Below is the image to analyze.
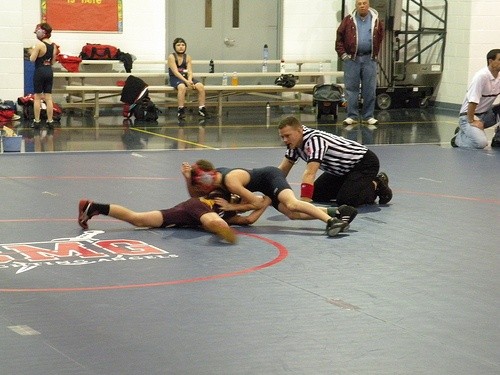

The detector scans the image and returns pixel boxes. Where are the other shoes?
[31,119,43,129]
[491,139,500,147]
[198,119,207,125]
[198,107,211,118]
[343,125,357,131]
[343,117,358,125]
[450,127,461,148]
[365,125,379,131]
[362,117,379,124]
[177,106,185,118]
[177,118,185,126]
[46,120,55,128]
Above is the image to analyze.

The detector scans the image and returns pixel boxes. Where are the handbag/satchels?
[274,73,296,88]
[80,42,119,61]
[135,98,159,121]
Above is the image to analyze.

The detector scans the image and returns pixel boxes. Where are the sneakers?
[338,204,358,224]
[326,218,350,237]
[78,200,93,230]
[375,172,393,204]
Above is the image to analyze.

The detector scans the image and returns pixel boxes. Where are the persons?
[30,23,57,130]
[335,0,384,124]
[276,116,393,206]
[167,37,209,120]
[181,160,357,236]
[77,190,272,243]
[451,50,500,149]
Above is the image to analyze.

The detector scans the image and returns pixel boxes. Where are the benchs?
[52,58,363,119]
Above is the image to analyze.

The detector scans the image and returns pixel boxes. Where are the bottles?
[231,71,239,86]
[280,60,285,73]
[265,102,271,116]
[208,59,214,73]
[263,45,269,60]
[262,61,267,72]
[318,62,323,72]
[222,72,227,85]
